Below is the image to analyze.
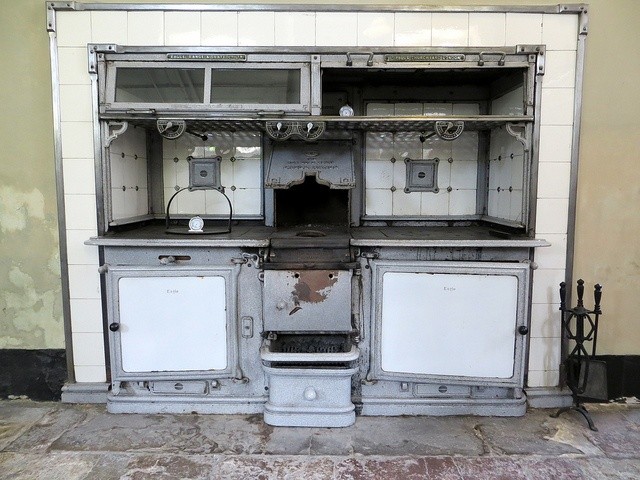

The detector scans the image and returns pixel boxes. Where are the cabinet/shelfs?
[371,255,526,396]
[106,262,246,388]
[316,58,539,125]
[96,53,313,124]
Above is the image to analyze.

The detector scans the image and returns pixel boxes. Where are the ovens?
[365,260,537,390]
[98,246,240,383]
